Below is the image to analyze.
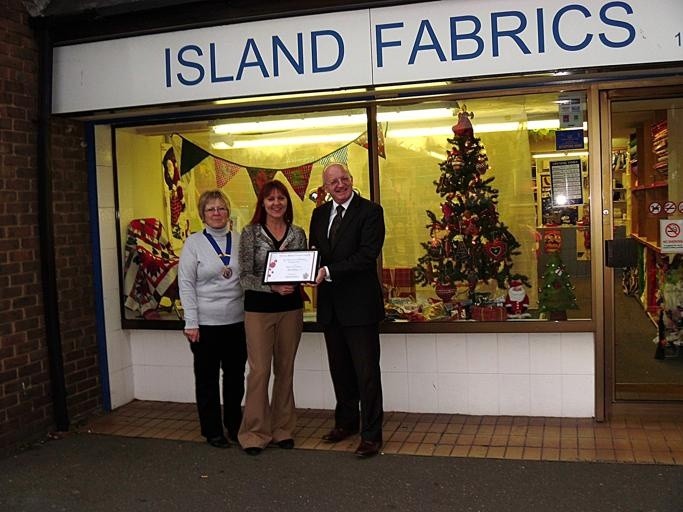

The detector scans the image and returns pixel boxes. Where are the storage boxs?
[382,268,416,302]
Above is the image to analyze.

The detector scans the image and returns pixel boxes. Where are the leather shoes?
[208,433,294,455]
[352,441,382,458]
[322,428,358,441]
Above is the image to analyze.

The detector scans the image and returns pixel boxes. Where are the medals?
[223,268,232,279]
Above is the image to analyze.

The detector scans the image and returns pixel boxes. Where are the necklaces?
[266,224,284,242]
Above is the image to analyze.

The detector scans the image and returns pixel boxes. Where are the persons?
[237,179,307,456]
[300,162,386,458]
[178,188,248,449]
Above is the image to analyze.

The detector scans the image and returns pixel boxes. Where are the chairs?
[121,217,185,318]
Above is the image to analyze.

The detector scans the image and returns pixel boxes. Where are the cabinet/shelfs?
[612,108,682,330]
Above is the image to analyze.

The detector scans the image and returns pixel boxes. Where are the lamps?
[207,100,461,136]
[532,150,589,159]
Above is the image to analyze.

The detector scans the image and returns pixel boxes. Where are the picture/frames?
[541,174,551,188]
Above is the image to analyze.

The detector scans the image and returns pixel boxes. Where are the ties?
[329,206,345,252]
